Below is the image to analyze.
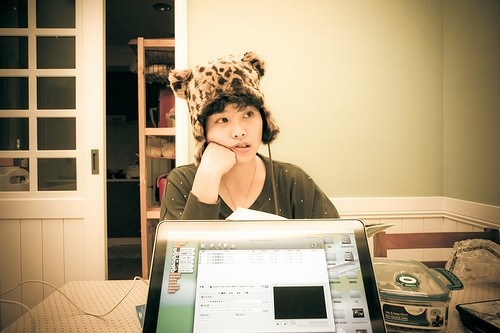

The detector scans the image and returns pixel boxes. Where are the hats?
[168,52,280,167]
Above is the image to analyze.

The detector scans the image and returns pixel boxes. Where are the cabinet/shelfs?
[106,65,161,122]
[128,36,176,278]
[107,182,141,238]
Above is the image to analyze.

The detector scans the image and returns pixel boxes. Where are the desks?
[0,281,500,333]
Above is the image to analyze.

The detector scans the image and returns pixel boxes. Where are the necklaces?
[222,159,257,210]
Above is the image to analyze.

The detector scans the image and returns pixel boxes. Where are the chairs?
[372,227,500,270]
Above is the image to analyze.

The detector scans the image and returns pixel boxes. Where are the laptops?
[140,217,388,333]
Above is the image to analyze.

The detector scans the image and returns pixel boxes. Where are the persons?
[158,50,340,220]
